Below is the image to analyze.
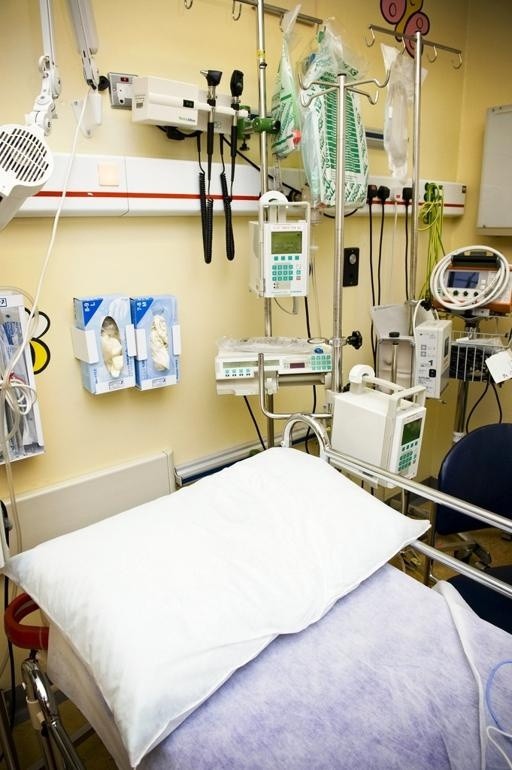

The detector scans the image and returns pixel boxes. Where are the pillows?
[0,445,433,770]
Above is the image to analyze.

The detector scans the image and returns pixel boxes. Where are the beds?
[0,408,512,770]
